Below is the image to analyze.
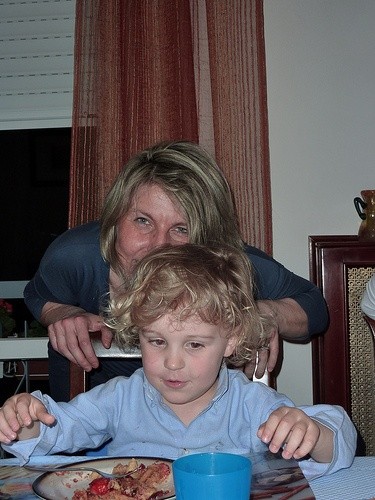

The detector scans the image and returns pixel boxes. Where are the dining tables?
[0,456,375,500]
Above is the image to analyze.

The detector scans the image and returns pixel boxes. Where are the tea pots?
[352,188,375,235]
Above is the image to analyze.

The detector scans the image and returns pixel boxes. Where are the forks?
[22,461,145,479]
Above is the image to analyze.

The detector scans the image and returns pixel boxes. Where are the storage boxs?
[69,338,270,399]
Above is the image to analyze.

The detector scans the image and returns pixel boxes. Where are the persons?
[0,243,357,480]
[23,141,329,402]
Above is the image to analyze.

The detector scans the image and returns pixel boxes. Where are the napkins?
[297,457,375,500]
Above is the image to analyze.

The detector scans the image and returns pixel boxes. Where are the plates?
[33,456,173,500]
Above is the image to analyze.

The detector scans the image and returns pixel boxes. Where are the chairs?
[0,281,49,394]
[308,234,375,457]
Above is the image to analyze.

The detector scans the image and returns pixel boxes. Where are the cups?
[170,451,255,498]
